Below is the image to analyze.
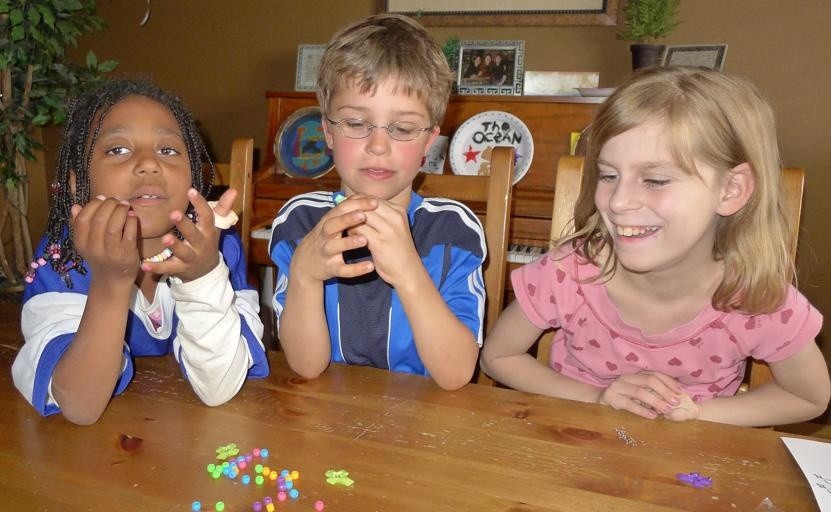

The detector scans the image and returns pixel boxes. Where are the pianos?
[251,90,610,346]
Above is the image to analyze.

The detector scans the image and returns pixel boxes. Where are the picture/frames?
[376,0,620,29]
[457,38,527,97]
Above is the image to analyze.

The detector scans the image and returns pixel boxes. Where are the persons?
[269,14,490,391]
[477,53,497,80]
[9,79,270,426]
[478,64,831,428]
[492,53,508,86]
[464,56,483,79]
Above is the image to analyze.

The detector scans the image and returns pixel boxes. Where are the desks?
[1,289,831,512]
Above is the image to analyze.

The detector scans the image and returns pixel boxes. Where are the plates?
[448,110,536,188]
[572,87,618,98]
[272,105,336,180]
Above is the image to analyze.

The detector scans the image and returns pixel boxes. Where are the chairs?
[340,146,516,386]
[537,155,807,431]
[197,137,255,282]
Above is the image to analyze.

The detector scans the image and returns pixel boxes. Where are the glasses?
[325,112,433,142]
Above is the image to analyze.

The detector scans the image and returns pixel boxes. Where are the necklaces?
[137,248,172,265]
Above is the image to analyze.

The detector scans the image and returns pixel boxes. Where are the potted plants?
[614,0,683,72]
[0,2,118,303]
